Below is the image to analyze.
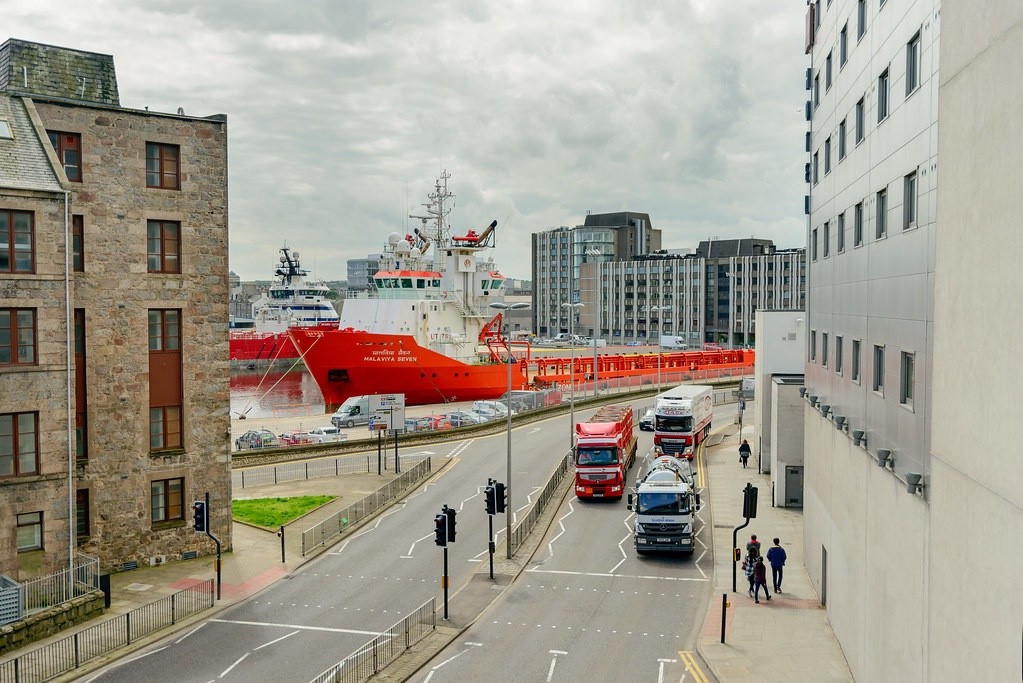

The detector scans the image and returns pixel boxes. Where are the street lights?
[651,306,667,393]
[490,303,530,559]
[561,302,585,448]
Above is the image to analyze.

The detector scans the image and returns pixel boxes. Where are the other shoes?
[778,588,782,593]
[748,590,752,598]
[767,595,771,600]
[755,601,759,603]
[774,587,777,593]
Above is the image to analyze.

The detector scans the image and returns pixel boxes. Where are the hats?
[744,439,747,442]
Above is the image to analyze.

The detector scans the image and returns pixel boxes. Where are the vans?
[498,390,545,410]
[331,396,368,428]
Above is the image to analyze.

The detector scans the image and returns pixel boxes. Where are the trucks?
[661,336,689,350]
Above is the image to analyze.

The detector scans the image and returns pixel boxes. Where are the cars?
[423,416,451,430]
[472,401,516,417]
[308,427,341,444]
[516,333,589,346]
[704,342,724,351]
[441,414,477,426]
[405,418,430,432]
[626,342,639,346]
[472,410,495,420]
[449,411,489,424]
[639,409,655,431]
[276,431,312,445]
[235,429,279,450]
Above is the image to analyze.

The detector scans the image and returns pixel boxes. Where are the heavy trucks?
[571,406,637,502]
[653,385,714,462]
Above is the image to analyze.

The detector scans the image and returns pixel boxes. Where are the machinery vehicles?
[627,455,700,558]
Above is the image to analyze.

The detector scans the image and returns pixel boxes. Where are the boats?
[285,169,755,405]
[229,239,339,368]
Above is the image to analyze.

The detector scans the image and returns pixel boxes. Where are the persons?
[744,549,757,597]
[754,556,771,603]
[579,451,590,459]
[766,538,787,593]
[746,535,761,557]
[738,439,751,468]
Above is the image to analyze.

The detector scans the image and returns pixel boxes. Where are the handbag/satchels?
[739,457,742,462]
[741,560,746,570]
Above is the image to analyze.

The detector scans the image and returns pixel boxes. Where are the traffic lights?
[191,501,206,533]
[484,483,508,515]
[434,514,448,548]
[443,508,457,543]
